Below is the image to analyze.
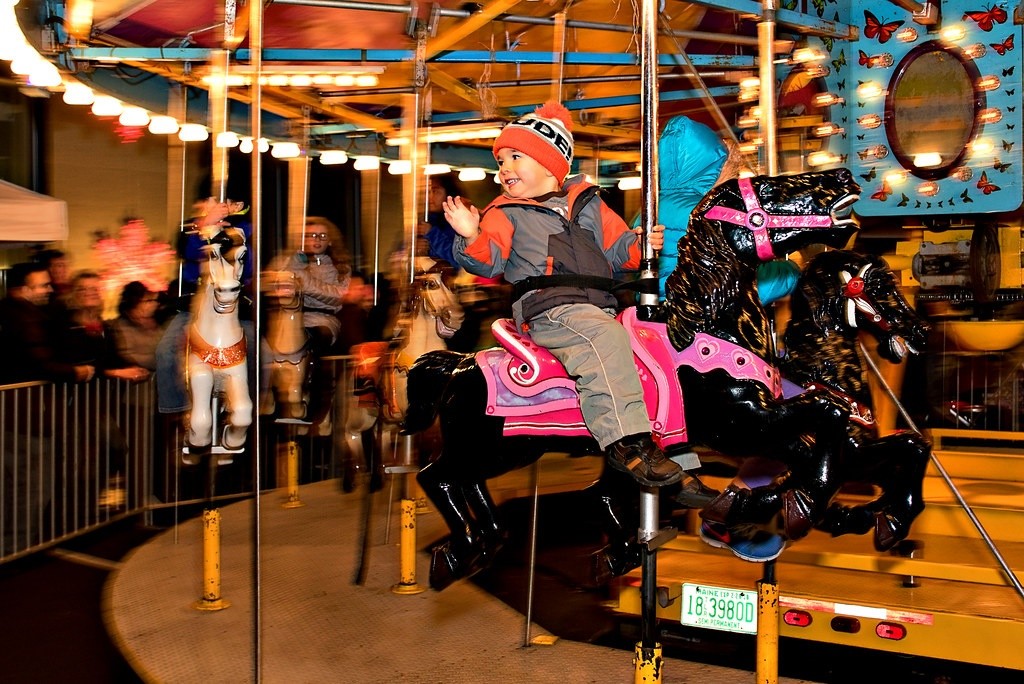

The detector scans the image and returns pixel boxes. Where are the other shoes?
[181,410,194,446]
[99,476,128,508]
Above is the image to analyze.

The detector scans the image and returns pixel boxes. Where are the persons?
[153,170,271,426]
[630,113,803,564]
[0,248,270,551]
[442,98,688,488]
[335,266,400,355]
[262,212,355,430]
[416,168,480,417]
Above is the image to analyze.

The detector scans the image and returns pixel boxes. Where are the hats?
[492,100,576,189]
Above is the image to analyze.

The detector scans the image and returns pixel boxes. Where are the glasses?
[304,233,329,241]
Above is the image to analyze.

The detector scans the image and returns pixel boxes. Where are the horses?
[396,168,863,594]
[661,248,935,554]
[257,249,468,495]
[155,228,254,466]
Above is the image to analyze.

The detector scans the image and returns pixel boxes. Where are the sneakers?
[659,467,717,507]
[408,496,430,515]
[602,431,682,487]
[699,515,787,562]
